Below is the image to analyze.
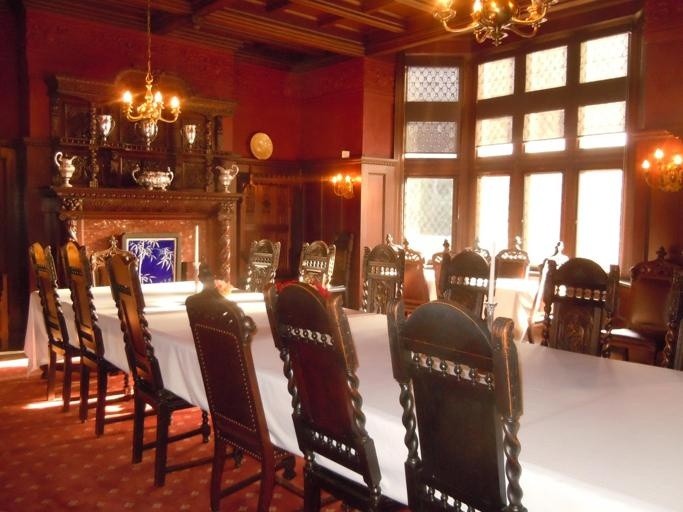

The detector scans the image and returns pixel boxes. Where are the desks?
[378,268,566,342]
[23,274,682,512]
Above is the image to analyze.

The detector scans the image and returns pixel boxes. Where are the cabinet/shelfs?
[46,71,239,188]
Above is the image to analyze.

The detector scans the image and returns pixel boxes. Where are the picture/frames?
[121,232,182,285]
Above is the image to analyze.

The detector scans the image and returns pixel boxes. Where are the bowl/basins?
[214,283,236,297]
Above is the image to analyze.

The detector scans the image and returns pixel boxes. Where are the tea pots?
[53,150,80,188]
[214,163,239,193]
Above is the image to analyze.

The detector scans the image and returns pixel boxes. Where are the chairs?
[358,242,407,320]
[329,237,353,311]
[519,242,570,344]
[245,237,282,294]
[104,248,242,487]
[600,245,682,368]
[184,288,297,512]
[665,308,665,311]
[539,257,619,360]
[439,250,490,326]
[388,237,531,278]
[61,237,156,438]
[386,299,526,512]
[28,241,131,413]
[262,282,382,512]
[296,240,336,293]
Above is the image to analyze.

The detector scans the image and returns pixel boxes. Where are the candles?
[194,223,199,263]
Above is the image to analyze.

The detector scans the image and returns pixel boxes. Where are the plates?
[249,132,274,160]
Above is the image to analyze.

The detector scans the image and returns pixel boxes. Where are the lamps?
[122,0,182,150]
[331,173,355,200]
[640,146,683,194]
[432,0,558,45]
